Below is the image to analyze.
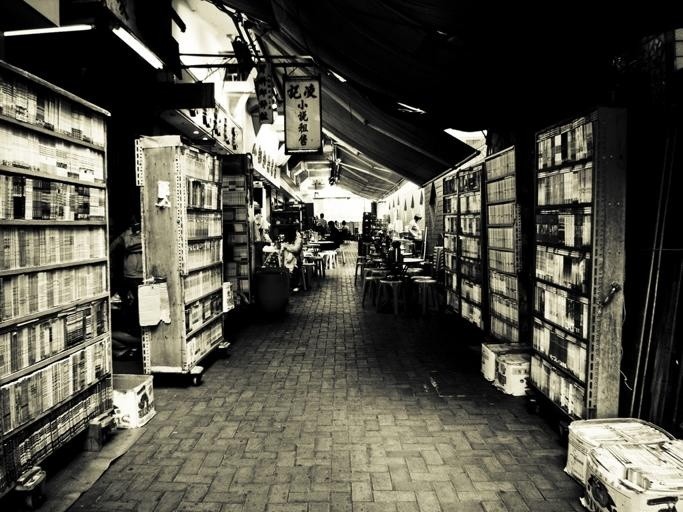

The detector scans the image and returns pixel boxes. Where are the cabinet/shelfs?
[215,150,255,318]
[444,105,629,421]
[3,58,116,505]
[133,133,229,382]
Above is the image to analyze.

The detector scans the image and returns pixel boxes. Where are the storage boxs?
[562,415,683,512]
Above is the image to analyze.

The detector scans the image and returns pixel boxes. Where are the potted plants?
[253,264,292,311]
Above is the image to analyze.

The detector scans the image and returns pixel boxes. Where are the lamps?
[111,23,167,71]
[3,24,96,37]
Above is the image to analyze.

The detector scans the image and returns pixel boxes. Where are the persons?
[255,211,351,295]
[112,211,143,351]
[367,212,425,271]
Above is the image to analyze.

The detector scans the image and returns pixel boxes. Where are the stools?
[293,231,440,321]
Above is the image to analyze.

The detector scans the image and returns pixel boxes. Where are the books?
[443,165,482,327]
[481,149,528,395]
[568,417,683,496]
[219,175,249,302]
[177,147,222,365]
[0,81,114,489]
[530,117,593,419]
